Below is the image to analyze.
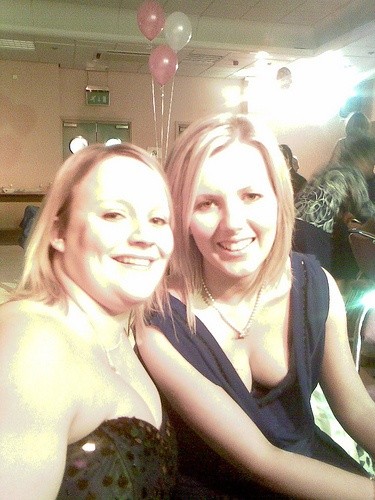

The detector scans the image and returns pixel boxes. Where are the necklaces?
[192,257,263,339]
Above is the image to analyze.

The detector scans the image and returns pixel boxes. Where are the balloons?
[149,44,177,86]
[137,1,164,44]
[163,11,194,54]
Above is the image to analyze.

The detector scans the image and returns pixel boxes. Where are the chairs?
[348,226,375,375]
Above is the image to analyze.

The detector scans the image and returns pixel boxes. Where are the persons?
[322,113,372,271]
[134,113,375,500]
[0,142,184,500]
[290,135,375,272]
[288,154,300,176]
[276,142,311,202]
